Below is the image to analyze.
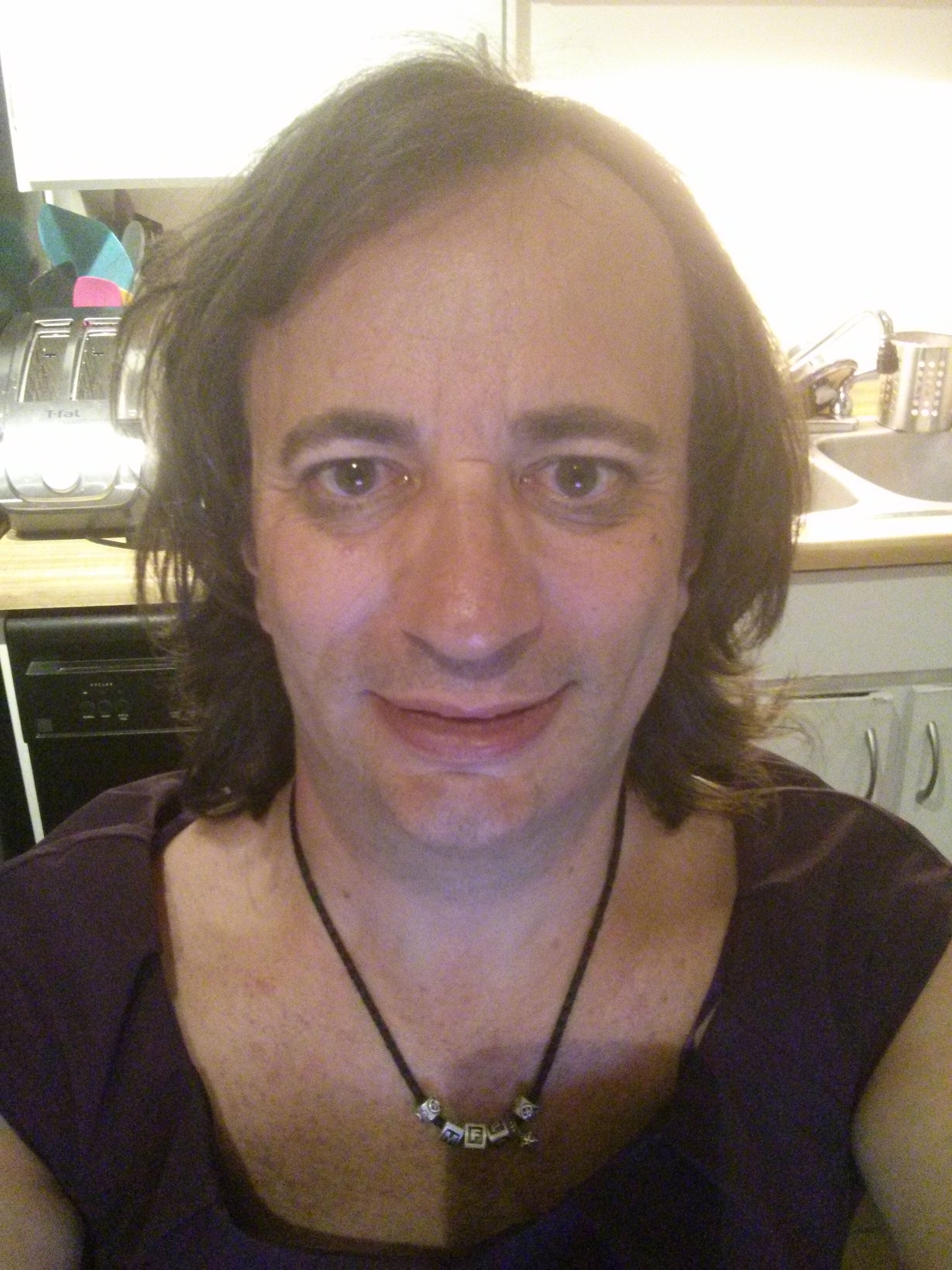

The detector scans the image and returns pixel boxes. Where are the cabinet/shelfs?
[2,1,520,193]
[727,684,952,864]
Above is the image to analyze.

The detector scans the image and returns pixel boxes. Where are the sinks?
[801,461,859,513]
[817,418,952,502]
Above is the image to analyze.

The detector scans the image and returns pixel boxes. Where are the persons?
[0,45,952,1270]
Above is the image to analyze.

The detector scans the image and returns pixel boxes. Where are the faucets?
[771,305,899,434]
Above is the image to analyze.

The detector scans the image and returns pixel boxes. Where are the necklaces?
[286,769,626,1147]
